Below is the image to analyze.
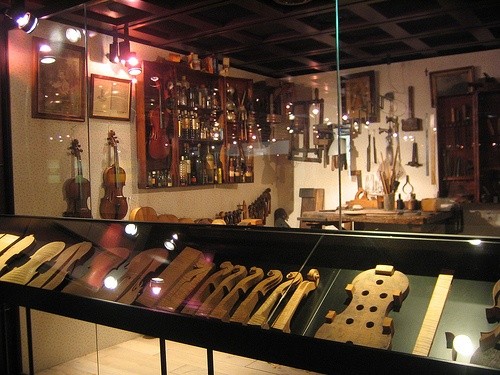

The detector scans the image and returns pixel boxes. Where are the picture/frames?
[340,70,379,123]
[91,74,132,121]
[31,36,85,122]
[429,66,474,107]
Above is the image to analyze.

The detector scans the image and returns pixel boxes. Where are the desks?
[302,200,457,234]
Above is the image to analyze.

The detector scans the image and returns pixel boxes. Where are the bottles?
[146,169,172,188]
[397,194,403,209]
[178,75,253,185]
[408,194,417,211]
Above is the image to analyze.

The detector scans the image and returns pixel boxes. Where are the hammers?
[401,86,419,132]
[408,143,421,167]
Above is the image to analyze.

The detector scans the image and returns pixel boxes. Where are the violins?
[99,129,129,219]
[146,77,170,159]
[64,139,94,219]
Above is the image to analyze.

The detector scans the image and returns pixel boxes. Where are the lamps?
[110,22,142,75]
[0,0,39,34]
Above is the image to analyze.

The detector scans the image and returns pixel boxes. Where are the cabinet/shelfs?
[435,92,500,204]
[136,59,254,189]
